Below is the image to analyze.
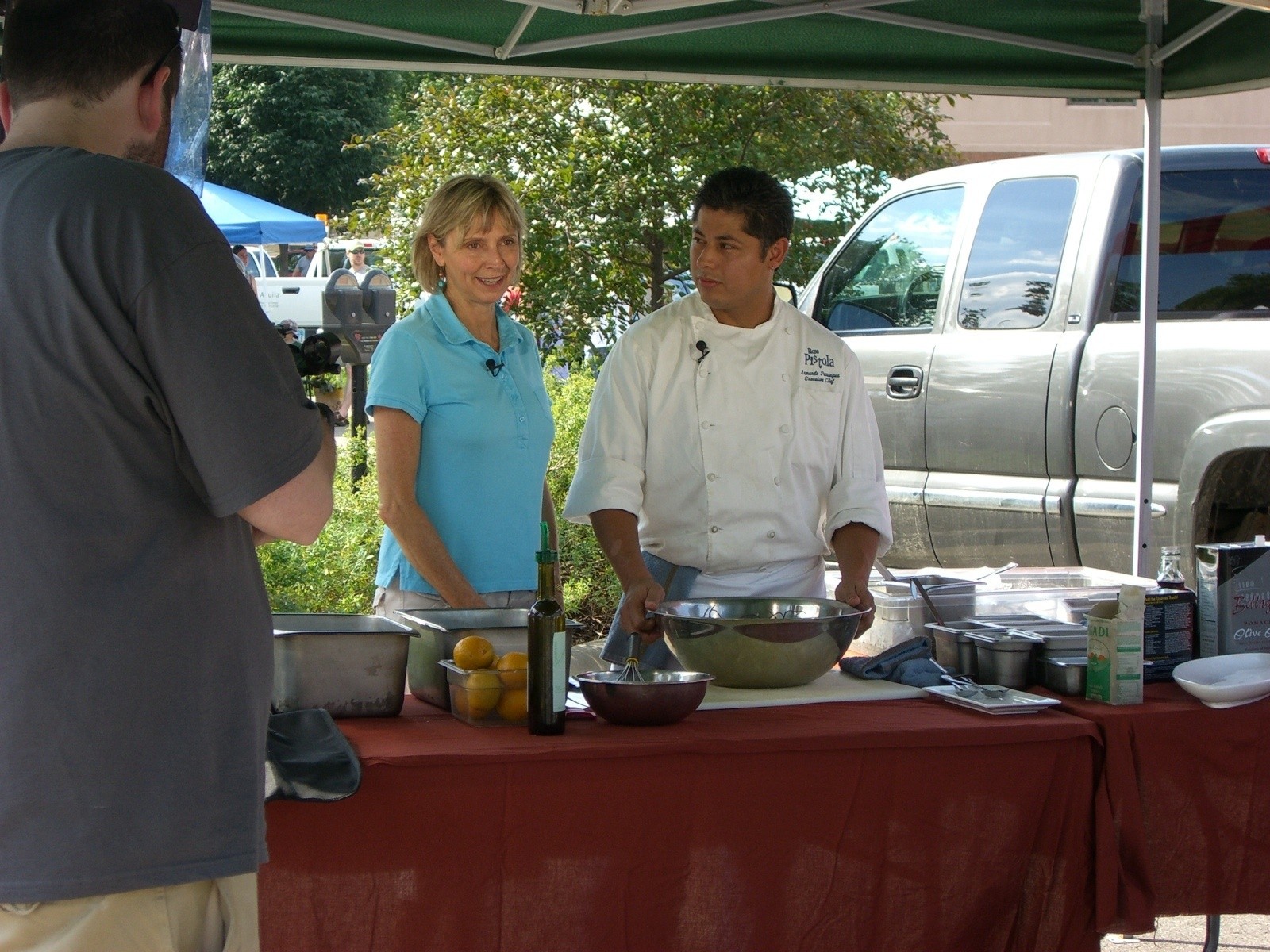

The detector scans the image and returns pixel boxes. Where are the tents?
[170,174,327,277]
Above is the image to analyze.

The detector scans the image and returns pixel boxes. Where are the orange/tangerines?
[453,636,529,720]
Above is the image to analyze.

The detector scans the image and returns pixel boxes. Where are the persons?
[364,173,555,626]
[232,244,258,299]
[562,165,893,671]
[0,0,337,952]
[275,319,308,377]
[292,244,317,277]
[342,240,375,289]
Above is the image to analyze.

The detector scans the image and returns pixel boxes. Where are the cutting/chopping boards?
[696,669,928,709]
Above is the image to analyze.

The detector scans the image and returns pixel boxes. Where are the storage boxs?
[1083,584,1148,703]
[254,277,337,325]
[269,612,421,716]
[395,605,586,710]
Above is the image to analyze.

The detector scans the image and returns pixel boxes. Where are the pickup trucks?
[770,141,1269,601]
[228,235,398,375]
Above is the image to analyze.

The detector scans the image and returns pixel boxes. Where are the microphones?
[486,359,496,376]
[696,340,706,357]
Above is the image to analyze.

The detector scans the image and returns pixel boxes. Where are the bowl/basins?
[574,671,717,725]
[646,597,872,689]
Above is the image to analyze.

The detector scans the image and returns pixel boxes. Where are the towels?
[263,708,361,803]
[839,634,975,690]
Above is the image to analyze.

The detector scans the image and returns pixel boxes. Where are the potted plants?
[304,373,344,412]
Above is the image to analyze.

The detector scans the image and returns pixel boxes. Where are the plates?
[1173,652,1270,710]
[922,683,1062,715]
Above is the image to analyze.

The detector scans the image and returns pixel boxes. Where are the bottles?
[528,520,568,736]
[1157,546,1185,590]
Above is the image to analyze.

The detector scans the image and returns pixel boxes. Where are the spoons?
[930,658,1010,698]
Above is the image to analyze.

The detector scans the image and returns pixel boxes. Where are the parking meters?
[320,264,401,491]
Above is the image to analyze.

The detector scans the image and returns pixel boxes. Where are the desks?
[259,661,1097,952]
[1025,683,1270,952]
